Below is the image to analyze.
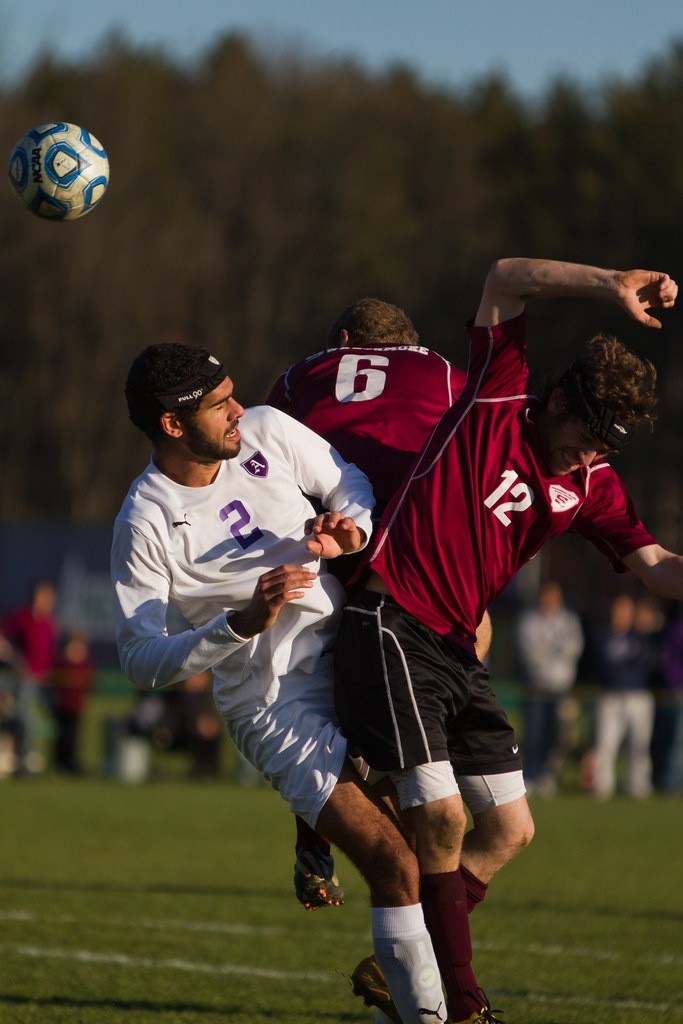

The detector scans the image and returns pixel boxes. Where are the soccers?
[7,121,110,221]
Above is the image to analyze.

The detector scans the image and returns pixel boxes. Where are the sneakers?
[293,852,344,910]
[448,987,503,1024]
[351,956,399,1024]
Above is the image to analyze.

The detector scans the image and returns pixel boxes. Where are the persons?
[1,583,92,779]
[108,259,683,1024]
